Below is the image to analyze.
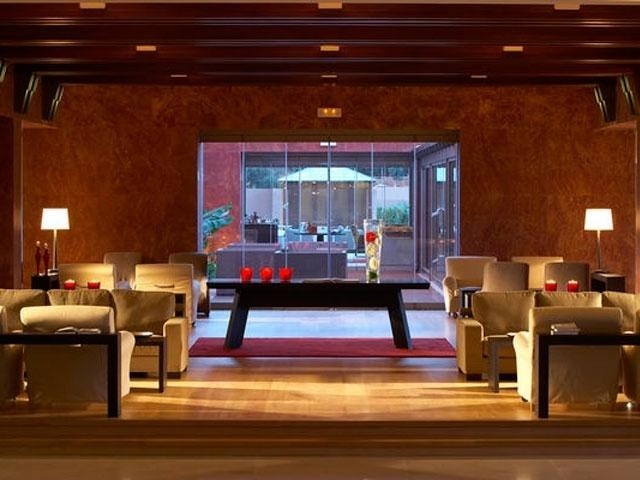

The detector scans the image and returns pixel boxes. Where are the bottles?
[34,241,42,276]
[42,242,50,276]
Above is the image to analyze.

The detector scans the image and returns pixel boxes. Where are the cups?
[544,282,557,291]
[299,221,318,234]
[568,281,579,292]
[64,282,76,290]
[362,219,383,279]
[240,268,252,280]
[87,281,100,289]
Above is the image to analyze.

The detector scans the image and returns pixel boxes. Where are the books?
[551,322,579,335]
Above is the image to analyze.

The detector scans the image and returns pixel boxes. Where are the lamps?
[40,207,71,272]
[585,207,613,272]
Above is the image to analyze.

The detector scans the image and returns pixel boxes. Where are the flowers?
[364,231,381,282]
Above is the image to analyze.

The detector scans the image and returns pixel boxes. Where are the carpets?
[189,336,456,358]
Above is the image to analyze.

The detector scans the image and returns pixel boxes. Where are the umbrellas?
[276,167,377,183]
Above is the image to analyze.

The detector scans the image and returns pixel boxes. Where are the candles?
[240,266,252,281]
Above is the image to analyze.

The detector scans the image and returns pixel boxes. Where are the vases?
[363,219,382,282]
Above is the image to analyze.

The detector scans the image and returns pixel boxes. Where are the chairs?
[351,226,366,256]
[442,256,639,420]
[0,249,208,418]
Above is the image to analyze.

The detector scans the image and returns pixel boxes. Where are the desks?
[207,278,429,348]
[293,227,346,242]
[245,223,278,245]
